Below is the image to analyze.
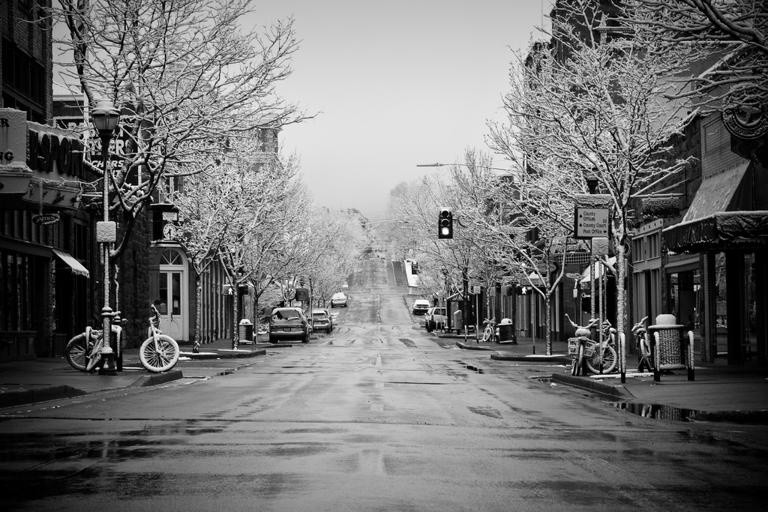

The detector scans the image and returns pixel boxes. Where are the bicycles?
[66,305,130,372]
[560,311,653,377]
[139,302,181,372]
[479,315,496,342]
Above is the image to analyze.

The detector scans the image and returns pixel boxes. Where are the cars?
[340,281,350,289]
[413,298,430,315]
[307,319,313,335]
[330,291,349,308]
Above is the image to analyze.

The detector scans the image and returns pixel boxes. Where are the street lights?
[85,94,126,372]
[416,163,537,356]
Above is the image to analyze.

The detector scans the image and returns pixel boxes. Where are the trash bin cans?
[496,318,514,344]
[239,319,254,344]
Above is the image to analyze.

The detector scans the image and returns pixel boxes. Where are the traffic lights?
[223,284,235,296]
[438,208,454,239]
[516,284,532,295]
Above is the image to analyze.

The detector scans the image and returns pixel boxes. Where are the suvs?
[422,306,448,331]
[309,308,334,335]
[268,307,310,344]
